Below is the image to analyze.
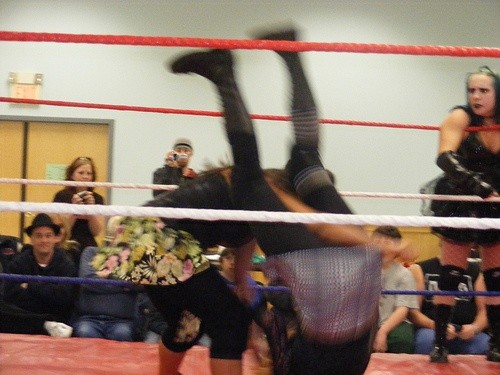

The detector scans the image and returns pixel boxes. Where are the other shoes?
[167,49,235,77]
[252,27,298,52]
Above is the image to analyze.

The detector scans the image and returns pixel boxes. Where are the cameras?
[174,154,188,163]
[77,192,87,202]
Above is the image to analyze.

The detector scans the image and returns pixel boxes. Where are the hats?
[172,136,194,154]
[25,212,61,237]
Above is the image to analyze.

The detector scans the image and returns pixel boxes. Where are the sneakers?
[50,322,73,339]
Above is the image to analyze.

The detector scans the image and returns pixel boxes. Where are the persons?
[167,26,381,375]
[429,66,500,361]
[0,144,490,375]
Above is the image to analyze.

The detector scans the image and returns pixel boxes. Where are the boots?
[428,303,453,364]
[482,302,500,362]
